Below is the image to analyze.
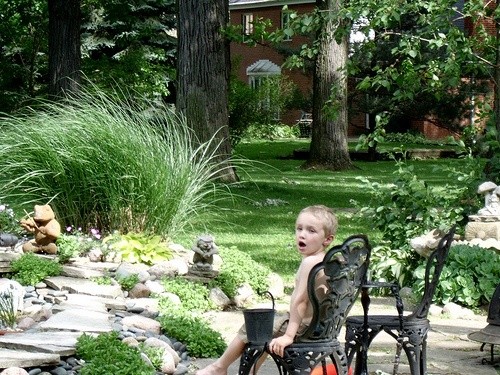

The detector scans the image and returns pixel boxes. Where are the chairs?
[344,224,456,375]
[239,235,372,375]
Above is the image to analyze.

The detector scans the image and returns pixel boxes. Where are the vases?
[241,308,276,346]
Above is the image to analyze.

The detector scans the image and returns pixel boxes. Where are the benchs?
[402,149,457,160]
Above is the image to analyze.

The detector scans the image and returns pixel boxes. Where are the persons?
[195,204,345,375]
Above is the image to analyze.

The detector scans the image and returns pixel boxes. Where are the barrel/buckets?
[242,288,276,346]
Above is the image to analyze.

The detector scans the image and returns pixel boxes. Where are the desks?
[321,282,404,375]
